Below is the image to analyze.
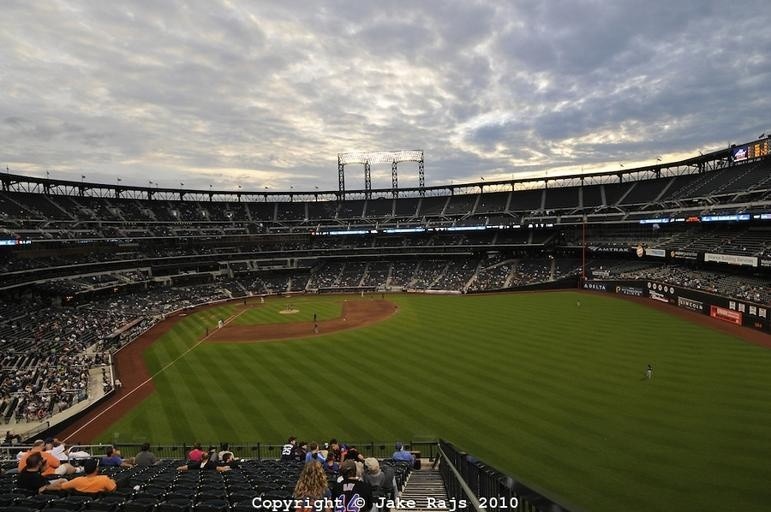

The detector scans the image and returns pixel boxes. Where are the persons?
[0,193,771,512]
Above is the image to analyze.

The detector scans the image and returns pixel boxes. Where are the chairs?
[0,457,410,512]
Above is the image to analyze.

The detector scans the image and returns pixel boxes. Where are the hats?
[364,457,379,471]
[341,459,357,477]
